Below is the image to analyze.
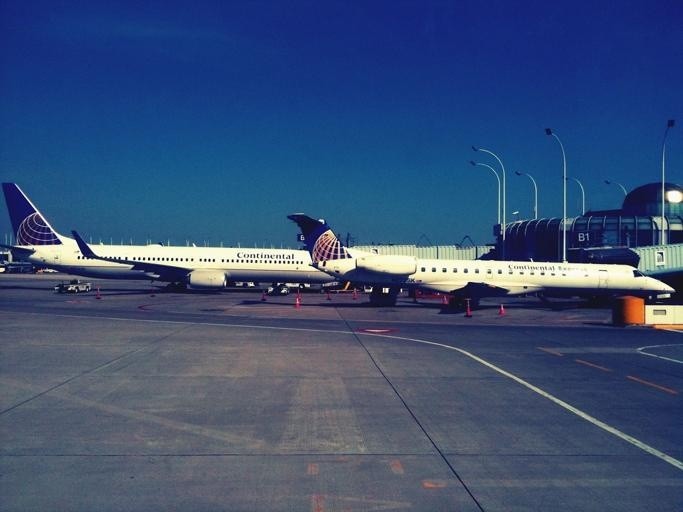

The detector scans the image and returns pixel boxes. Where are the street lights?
[470,145,506,241]
[562,176,585,216]
[661,119,674,247]
[604,180,627,197]
[544,127,567,261]
[514,170,537,220]
